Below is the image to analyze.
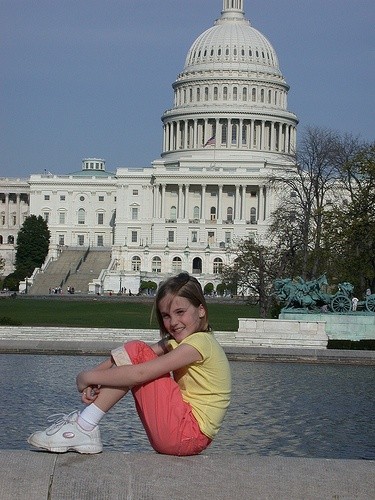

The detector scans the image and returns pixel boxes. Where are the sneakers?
[27,409,103,454]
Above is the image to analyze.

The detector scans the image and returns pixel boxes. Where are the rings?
[83,392,86,395]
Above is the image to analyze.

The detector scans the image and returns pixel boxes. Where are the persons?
[27,273,231,455]
[49,285,244,298]
